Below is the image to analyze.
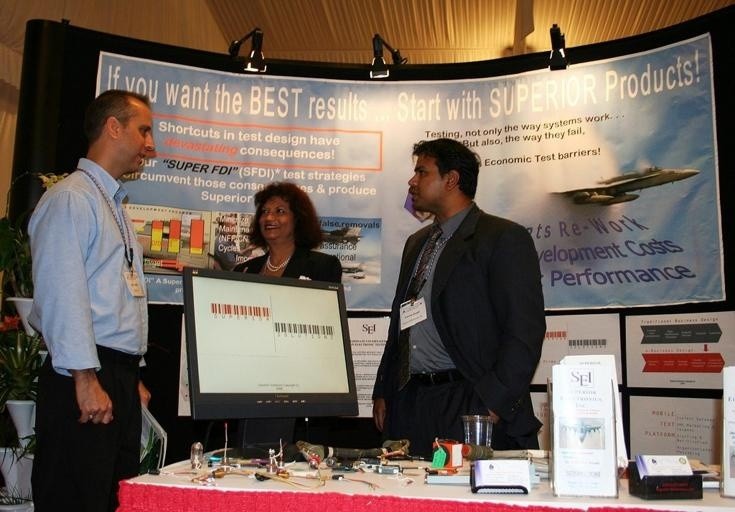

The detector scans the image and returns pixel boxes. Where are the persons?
[230,181,344,461]
[371,137,548,461]
[24,91,157,512]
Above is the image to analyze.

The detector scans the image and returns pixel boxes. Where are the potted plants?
[1,213,43,511]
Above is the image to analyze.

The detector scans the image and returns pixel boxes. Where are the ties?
[393,223,444,393]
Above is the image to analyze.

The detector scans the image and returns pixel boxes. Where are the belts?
[404,368,468,389]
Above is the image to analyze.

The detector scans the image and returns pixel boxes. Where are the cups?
[461,412,494,447]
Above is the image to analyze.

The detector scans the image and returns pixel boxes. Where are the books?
[139,402,167,475]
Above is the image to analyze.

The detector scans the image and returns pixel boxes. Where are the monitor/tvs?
[183,266,358,462]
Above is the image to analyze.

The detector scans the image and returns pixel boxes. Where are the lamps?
[543,21,572,75]
[365,31,407,78]
[228,23,271,76]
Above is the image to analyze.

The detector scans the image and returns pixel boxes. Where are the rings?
[88,414,94,419]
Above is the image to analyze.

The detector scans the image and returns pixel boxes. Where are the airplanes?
[323,225,362,244]
[550,166,700,206]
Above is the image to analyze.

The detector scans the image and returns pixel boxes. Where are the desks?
[117,444,735,512]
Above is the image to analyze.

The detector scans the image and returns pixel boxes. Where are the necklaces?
[266,254,291,272]
